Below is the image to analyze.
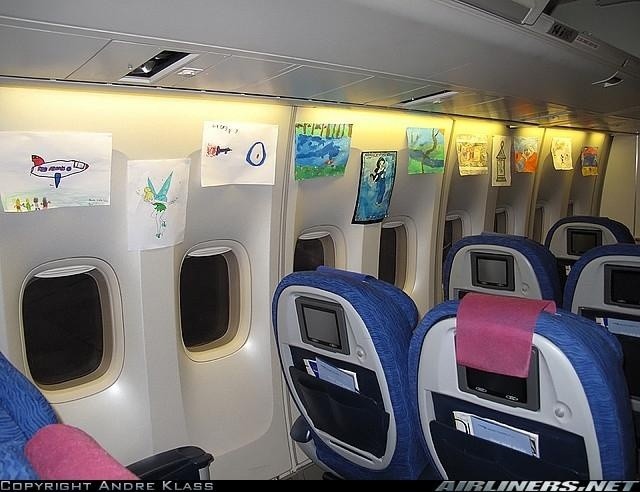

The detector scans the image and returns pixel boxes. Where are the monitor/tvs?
[295,298,348,355]
[456,335,542,411]
[604,263,640,309]
[567,228,601,255]
[470,252,515,293]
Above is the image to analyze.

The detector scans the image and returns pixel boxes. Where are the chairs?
[564,246,640,419]
[408,292,640,479]
[442,231,565,307]
[0,353,211,479]
[270,261,432,480]
[545,216,633,280]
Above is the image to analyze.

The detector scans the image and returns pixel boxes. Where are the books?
[303,358,360,393]
[452,410,541,459]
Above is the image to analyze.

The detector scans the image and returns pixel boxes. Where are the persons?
[144,187,168,238]
[369,157,388,208]
